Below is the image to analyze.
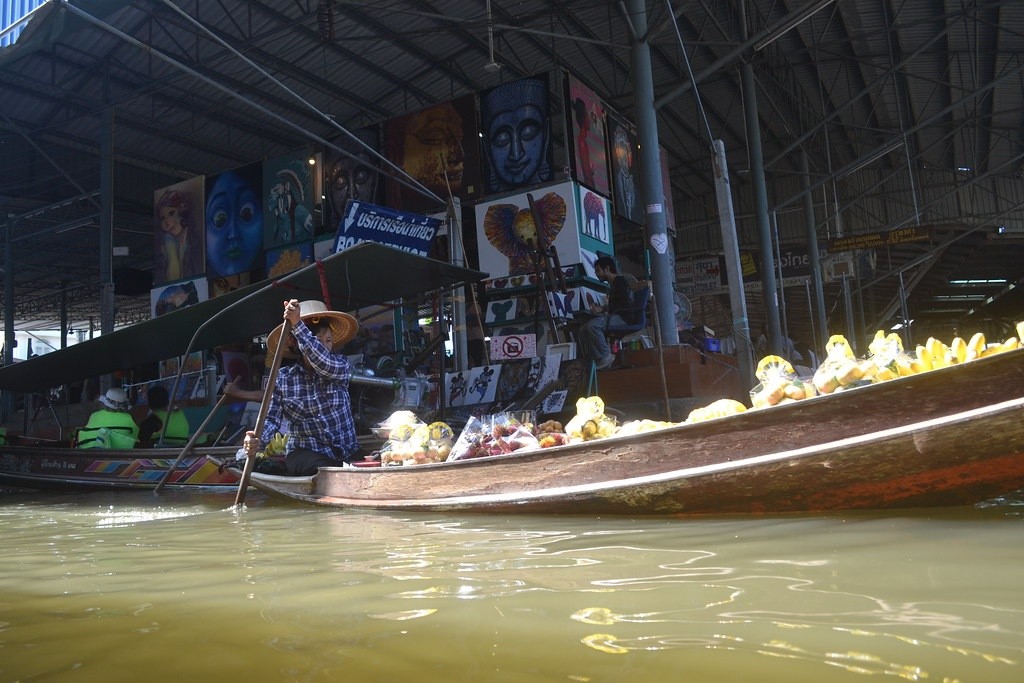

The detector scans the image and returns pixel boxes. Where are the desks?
[590,344,739,404]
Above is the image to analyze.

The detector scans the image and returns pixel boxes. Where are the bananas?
[881,333,1018,381]
[265,432,289,458]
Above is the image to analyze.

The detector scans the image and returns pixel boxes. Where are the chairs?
[605,287,651,353]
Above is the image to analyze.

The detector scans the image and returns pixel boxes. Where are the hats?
[99,388,132,409]
[266,300,359,359]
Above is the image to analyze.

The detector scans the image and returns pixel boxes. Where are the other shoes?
[596,353,615,370]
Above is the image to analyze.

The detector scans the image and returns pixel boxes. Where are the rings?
[290,305,296,311]
[247,442,253,449]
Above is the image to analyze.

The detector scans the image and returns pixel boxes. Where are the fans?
[673,291,692,327]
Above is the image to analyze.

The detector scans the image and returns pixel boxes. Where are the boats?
[206,342,1024,522]
[0,239,491,502]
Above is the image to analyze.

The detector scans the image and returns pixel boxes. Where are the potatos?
[539,420,563,433]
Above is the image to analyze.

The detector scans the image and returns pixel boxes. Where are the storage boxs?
[678,325,715,337]
[704,338,720,352]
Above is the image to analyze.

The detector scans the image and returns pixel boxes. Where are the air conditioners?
[829,259,856,281]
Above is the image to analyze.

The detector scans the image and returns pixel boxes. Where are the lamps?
[752,0,834,53]
[54,217,97,234]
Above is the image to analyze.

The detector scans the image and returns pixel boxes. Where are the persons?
[243,300,362,476]
[579,257,640,370]
[75,387,140,448]
[757,322,820,369]
[223,382,265,402]
[135,386,189,444]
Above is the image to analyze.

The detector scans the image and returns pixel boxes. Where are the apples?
[761,359,885,407]
[381,445,449,466]
